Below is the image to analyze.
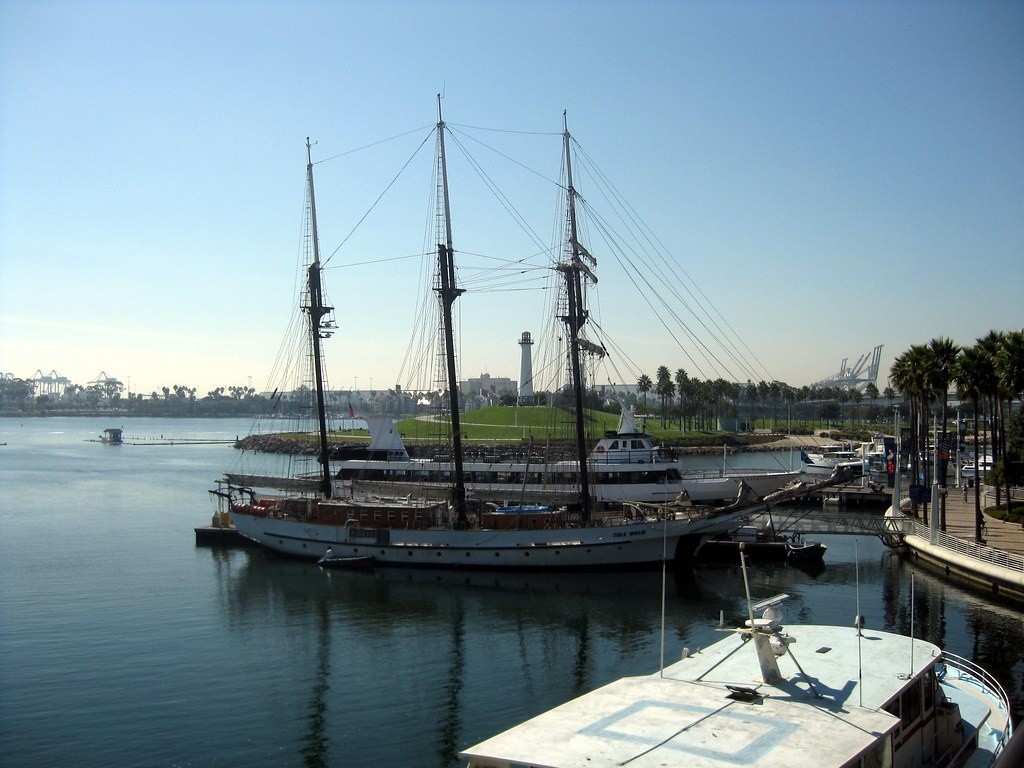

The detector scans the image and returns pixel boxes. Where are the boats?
[441,534,1020,766]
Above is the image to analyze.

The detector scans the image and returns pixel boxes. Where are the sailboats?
[208,93,996,583]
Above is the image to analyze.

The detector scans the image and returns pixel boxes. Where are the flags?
[882,437,895,489]
[900,427,911,456]
[959,421,968,435]
[801,449,815,464]
[937,431,952,487]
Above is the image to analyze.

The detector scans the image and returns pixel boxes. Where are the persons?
[980,509,985,529]
[961,482,968,499]
